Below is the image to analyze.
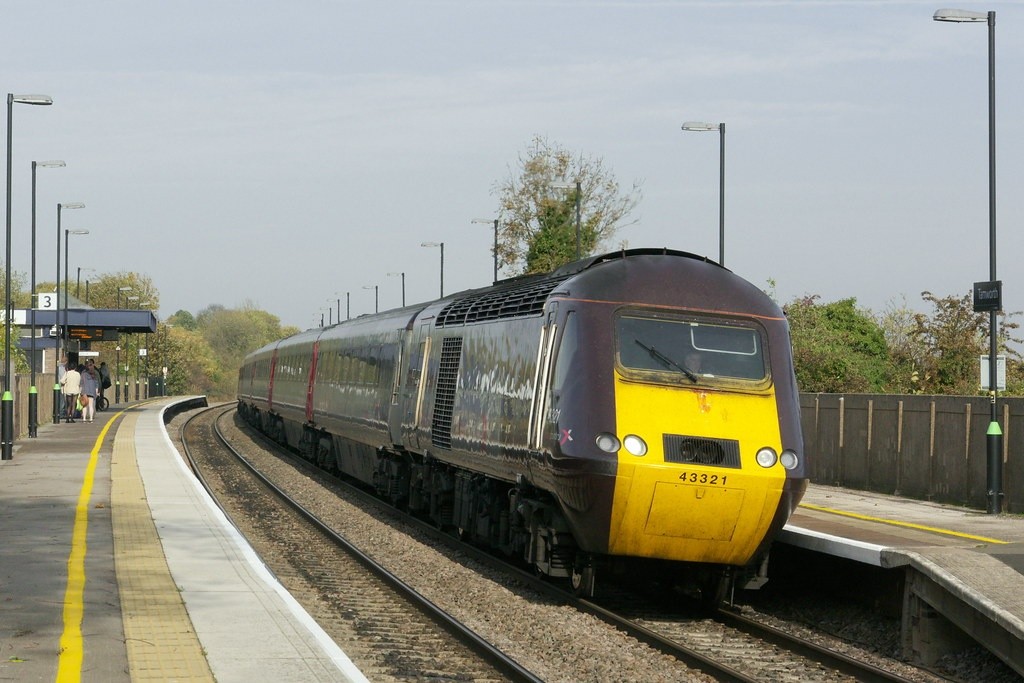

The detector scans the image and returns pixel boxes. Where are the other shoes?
[66,420,69,423]
[84,421,87,424]
[71,420,75,423]
[90,421,93,423]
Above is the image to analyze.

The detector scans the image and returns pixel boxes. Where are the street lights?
[548,180,580,260]
[387,273,405,308]
[683,120,725,267]
[2,94,54,459]
[63,229,89,357]
[156,320,174,397]
[29,160,66,438]
[135,303,150,400]
[932,7,1005,515]
[471,217,498,282]
[53,202,86,423]
[116,287,132,405]
[76,265,102,304]
[421,241,444,299]
[362,285,378,313]
[125,296,139,402]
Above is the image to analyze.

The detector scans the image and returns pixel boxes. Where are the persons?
[59,357,68,418]
[80,359,101,423]
[684,352,704,373]
[97,362,109,411]
[61,362,80,423]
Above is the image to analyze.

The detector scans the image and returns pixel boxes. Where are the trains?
[237,247,806,608]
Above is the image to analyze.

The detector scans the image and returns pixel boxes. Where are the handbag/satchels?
[76,394,83,411]
[80,393,89,407]
